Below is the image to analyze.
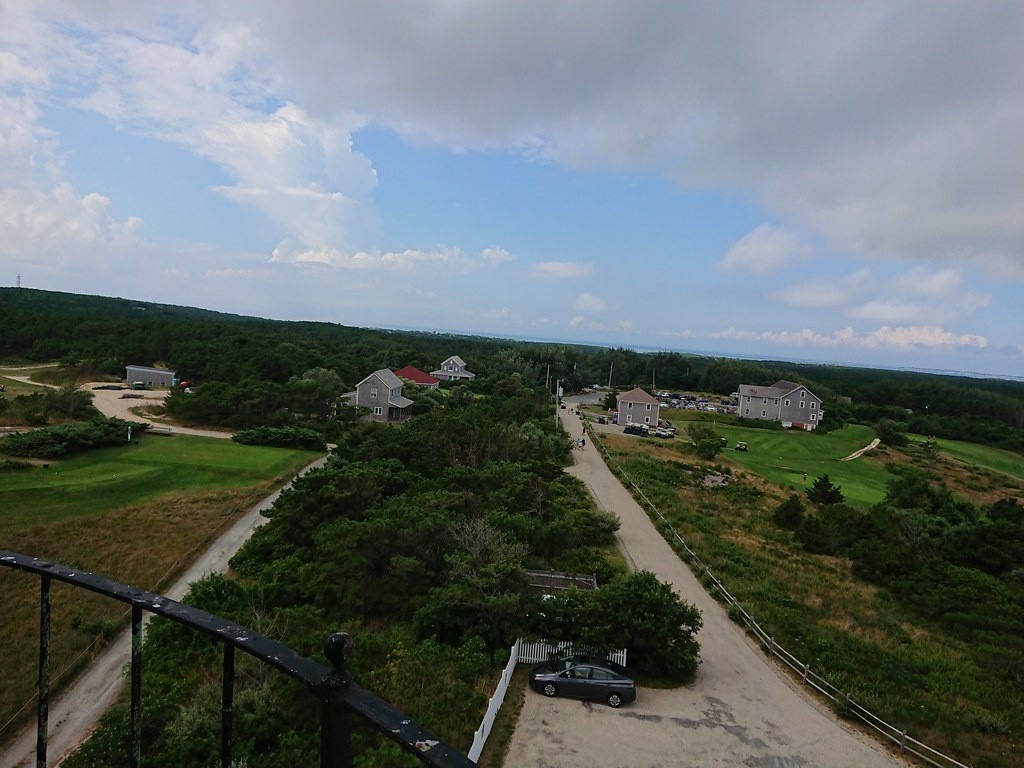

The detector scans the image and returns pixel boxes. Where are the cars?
[155,363,169,371]
[184,388,193,394]
[130,381,144,390]
[656,391,739,414]
[529,655,637,708]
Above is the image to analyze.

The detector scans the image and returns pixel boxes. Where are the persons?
[804,474,807,484]
[574,438,586,451]
[582,427,586,435]
